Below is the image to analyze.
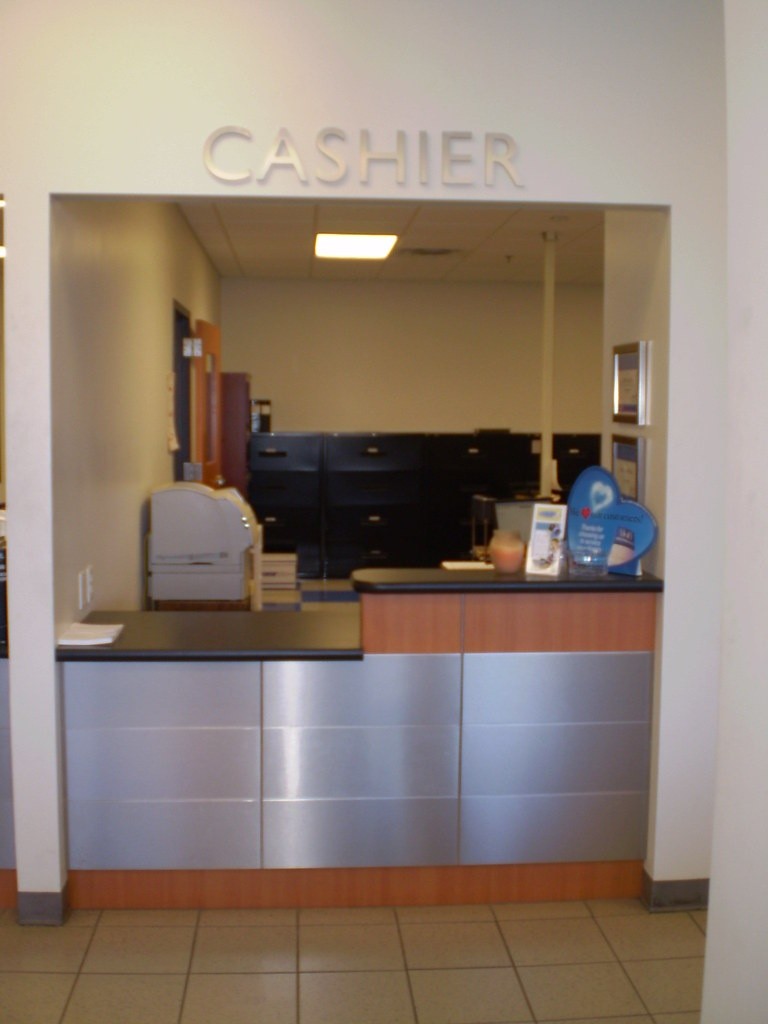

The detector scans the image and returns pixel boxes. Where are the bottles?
[490,529,525,574]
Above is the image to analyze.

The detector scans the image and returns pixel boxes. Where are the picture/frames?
[611,341,645,427]
[611,433,645,503]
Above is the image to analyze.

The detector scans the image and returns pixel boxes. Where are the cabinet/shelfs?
[248,432,600,580]
[220,369,253,497]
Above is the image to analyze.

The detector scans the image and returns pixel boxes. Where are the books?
[58,622,124,646]
